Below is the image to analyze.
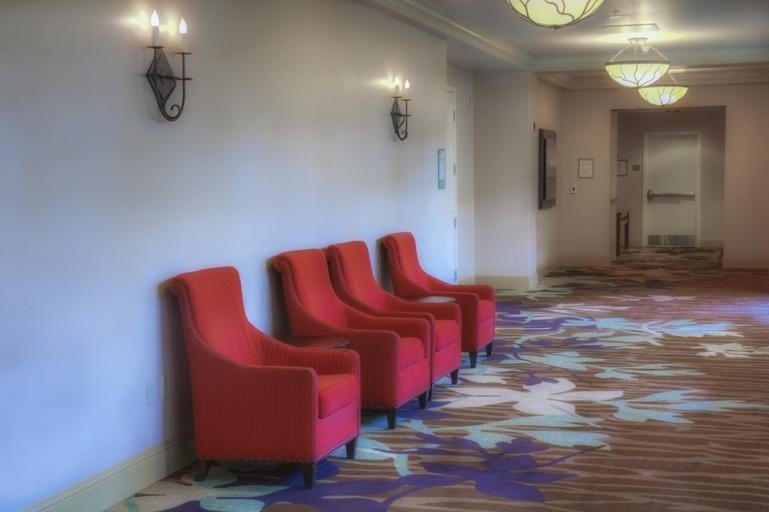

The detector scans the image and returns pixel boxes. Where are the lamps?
[147,9,189,123]
[390,78,410,141]
[505,0,604,30]
[605,37,689,107]
